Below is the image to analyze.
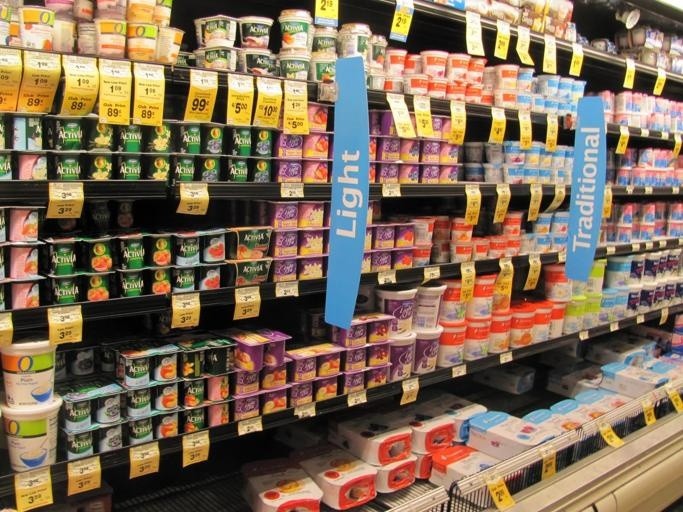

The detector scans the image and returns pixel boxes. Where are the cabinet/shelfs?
[0,1,682,510]
